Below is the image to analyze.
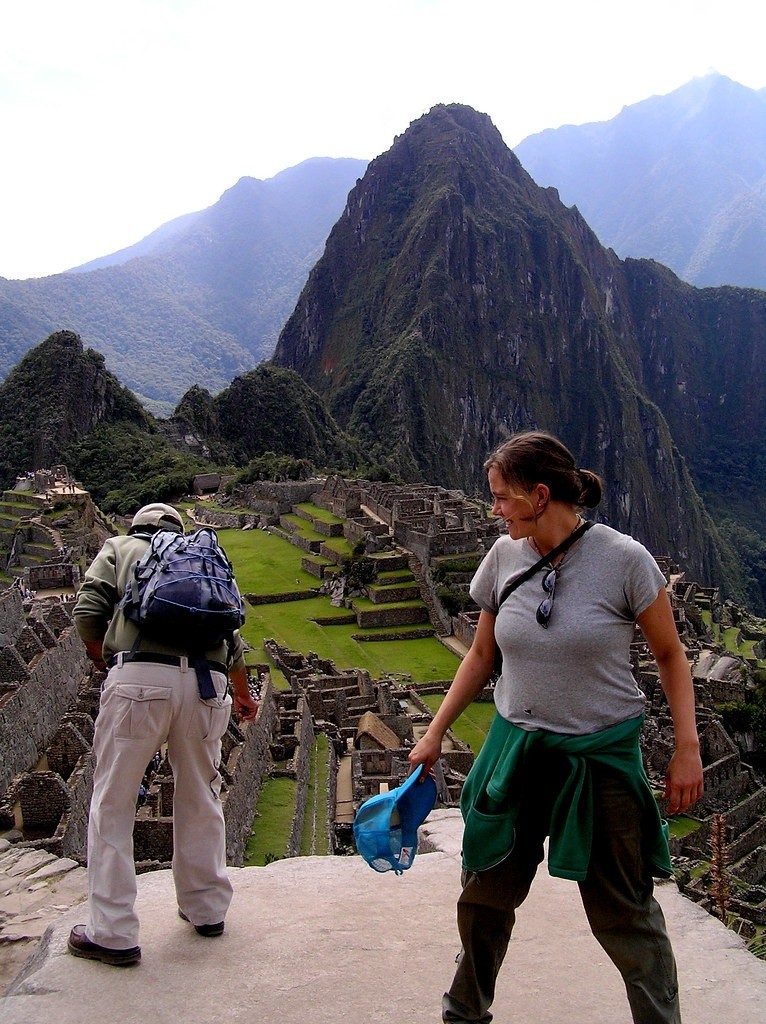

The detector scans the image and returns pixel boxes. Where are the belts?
[111,652,228,676]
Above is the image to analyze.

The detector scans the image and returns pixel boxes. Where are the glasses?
[536,569,557,629]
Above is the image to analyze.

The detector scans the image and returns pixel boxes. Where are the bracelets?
[86,651,103,663]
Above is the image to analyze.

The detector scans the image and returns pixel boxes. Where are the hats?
[353,764,437,876]
[127,503,185,534]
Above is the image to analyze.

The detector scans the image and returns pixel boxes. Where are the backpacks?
[114,526,246,650]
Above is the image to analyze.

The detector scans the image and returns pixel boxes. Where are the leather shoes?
[68,924,142,964]
[179,907,225,936]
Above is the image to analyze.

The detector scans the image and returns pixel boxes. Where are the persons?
[66,502,258,963]
[18,588,37,599]
[409,432,705,1024]
[137,751,161,805]
[60,592,73,601]
[246,673,262,701]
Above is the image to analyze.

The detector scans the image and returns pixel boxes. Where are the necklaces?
[533,514,581,569]
